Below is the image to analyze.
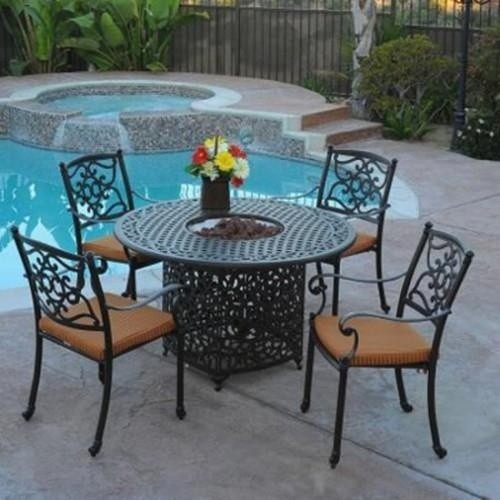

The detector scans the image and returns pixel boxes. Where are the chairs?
[300,221,474,468]
[11,226,185,457]
[59,149,162,304]
[271,145,397,315]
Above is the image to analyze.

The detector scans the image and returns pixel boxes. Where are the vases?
[201,174,229,210]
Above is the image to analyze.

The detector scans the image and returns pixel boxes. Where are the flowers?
[185,135,250,187]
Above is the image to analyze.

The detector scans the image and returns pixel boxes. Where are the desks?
[114,198,357,391]
